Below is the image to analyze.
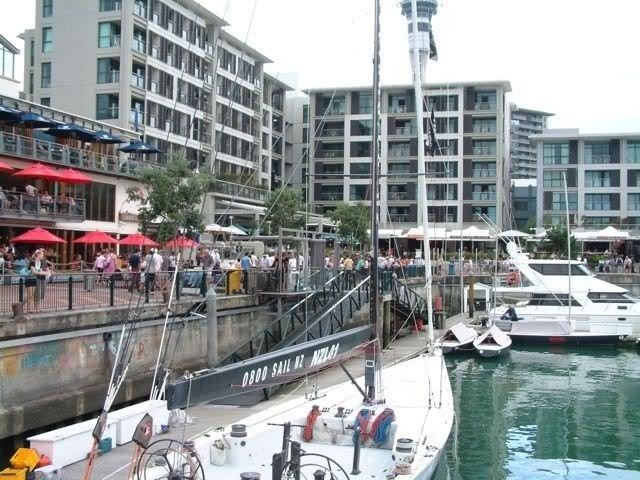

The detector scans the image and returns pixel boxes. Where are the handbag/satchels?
[19,260,35,276]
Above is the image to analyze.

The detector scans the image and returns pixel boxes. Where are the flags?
[426,119,438,158]
[430,106,437,133]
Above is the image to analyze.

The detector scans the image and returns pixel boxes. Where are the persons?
[73,247,183,303]
[0,182,81,217]
[325,255,334,267]
[448,254,513,272]
[236,242,304,292]
[597,249,636,273]
[377,254,426,278]
[1,242,54,311]
[339,253,370,284]
[196,247,221,297]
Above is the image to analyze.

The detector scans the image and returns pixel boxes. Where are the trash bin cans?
[434,312,447,329]
[82,269,98,292]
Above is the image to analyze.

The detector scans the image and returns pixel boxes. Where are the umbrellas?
[0,104,164,200]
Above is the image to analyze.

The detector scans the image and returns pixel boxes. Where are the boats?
[473,323,513,357]
[436,322,477,355]
[80,0,457,480]
[476,172,640,346]
